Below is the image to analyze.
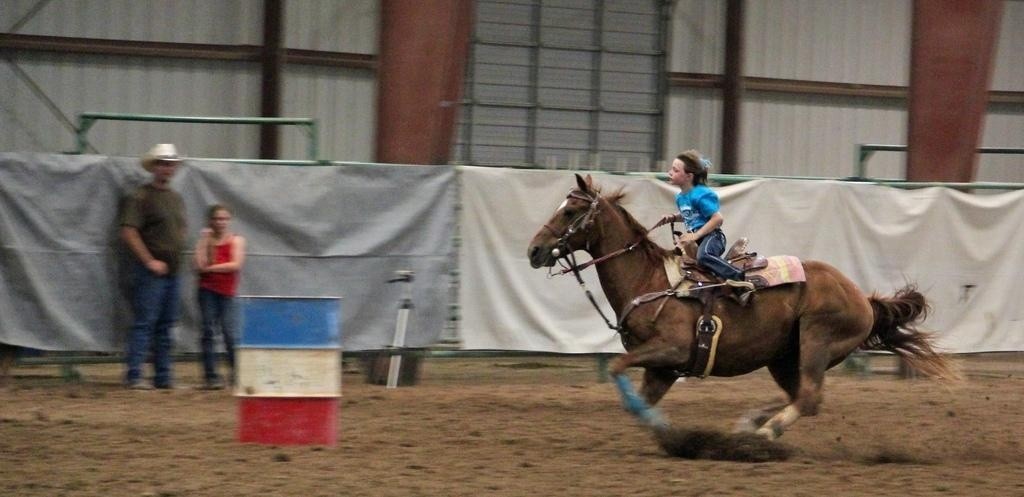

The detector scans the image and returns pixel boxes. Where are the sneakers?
[731,273,751,307]
[126,380,224,391]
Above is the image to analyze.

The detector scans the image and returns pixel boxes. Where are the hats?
[143,144,186,173]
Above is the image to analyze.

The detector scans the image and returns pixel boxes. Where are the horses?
[525,171,962,444]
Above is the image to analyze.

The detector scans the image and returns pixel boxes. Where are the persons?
[668,150,752,308]
[193,205,246,388]
[119,143,191,391]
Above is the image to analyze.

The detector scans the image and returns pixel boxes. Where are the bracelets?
[671,214,677,222]
